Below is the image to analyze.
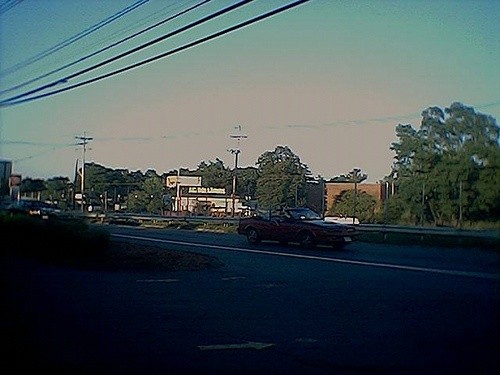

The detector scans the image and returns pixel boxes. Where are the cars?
[4,198,58,223]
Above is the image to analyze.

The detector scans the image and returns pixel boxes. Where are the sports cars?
[237,206,362,251]
